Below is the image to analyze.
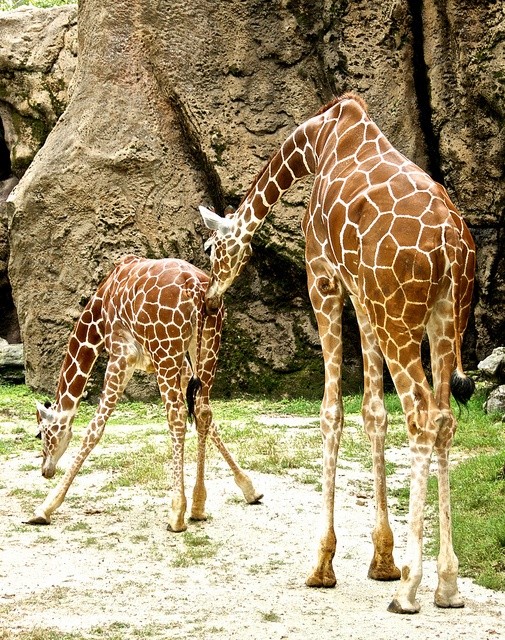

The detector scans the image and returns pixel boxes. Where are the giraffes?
[21,255,265,534]
[198,93,478,616]
